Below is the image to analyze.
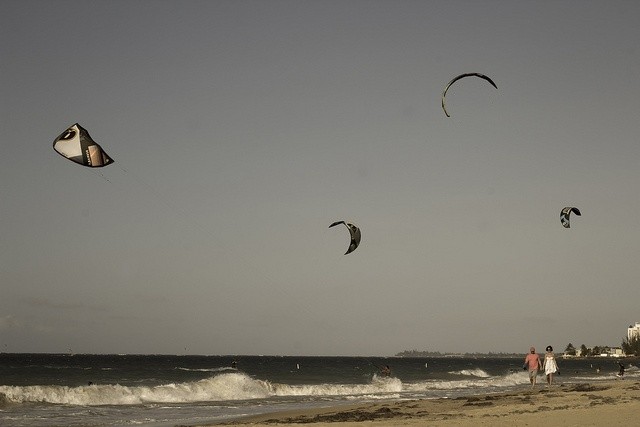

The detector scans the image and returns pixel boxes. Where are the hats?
[530,347,535,351]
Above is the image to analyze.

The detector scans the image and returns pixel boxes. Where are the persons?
[525,347,542,388]
[616,361,625,380]
[543,345,559,385]
[381,365,391,378]
